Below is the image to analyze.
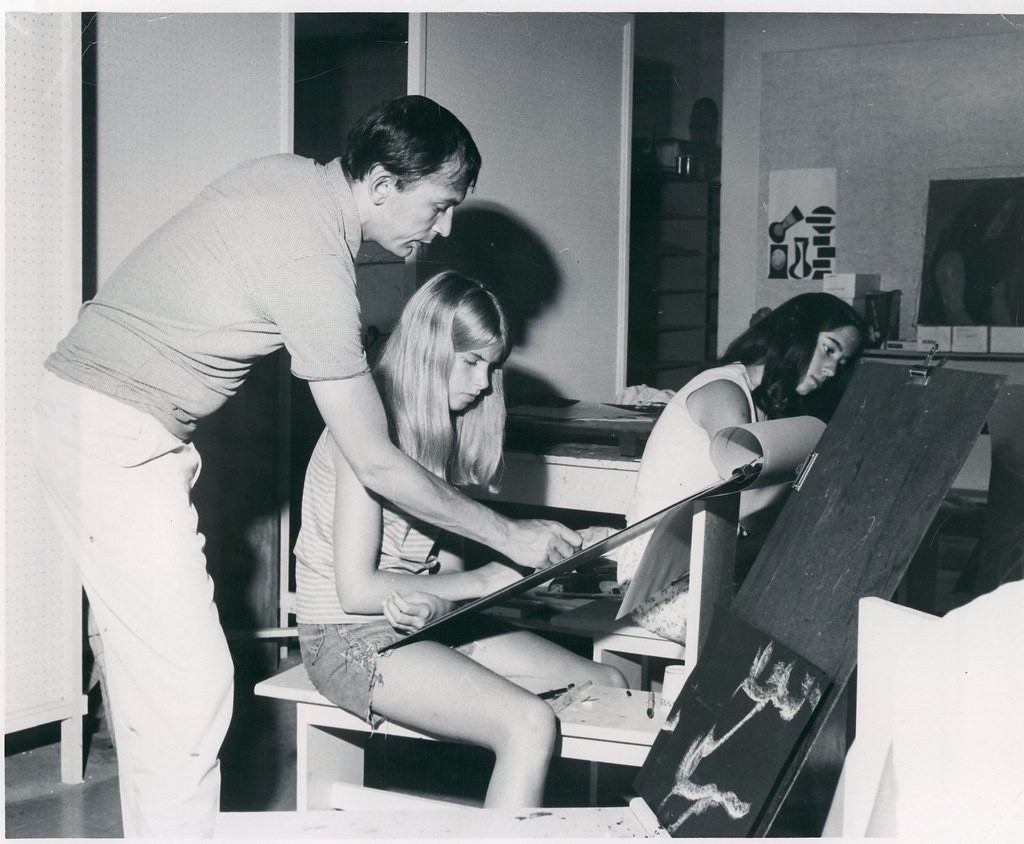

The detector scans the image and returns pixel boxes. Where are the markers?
[647,691,655,719]
[537,682,575,702]
[552,680,593,712]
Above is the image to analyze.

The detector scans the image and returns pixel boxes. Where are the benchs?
[252,663,662,811]
[510,593,686,692]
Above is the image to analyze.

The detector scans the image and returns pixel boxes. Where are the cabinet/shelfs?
[625,172,722,387]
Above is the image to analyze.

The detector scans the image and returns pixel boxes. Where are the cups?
[656,663,685,732]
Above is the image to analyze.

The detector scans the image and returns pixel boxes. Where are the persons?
[29,94,583,838]
[292,270,630,809]
[617,292,866,648]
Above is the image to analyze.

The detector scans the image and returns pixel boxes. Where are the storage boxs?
[951,324,990,352]
[823,271,880,298]
[990,325,1024,353]
[916,325,951,352]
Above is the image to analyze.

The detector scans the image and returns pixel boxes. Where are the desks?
[475,445,642,514]
